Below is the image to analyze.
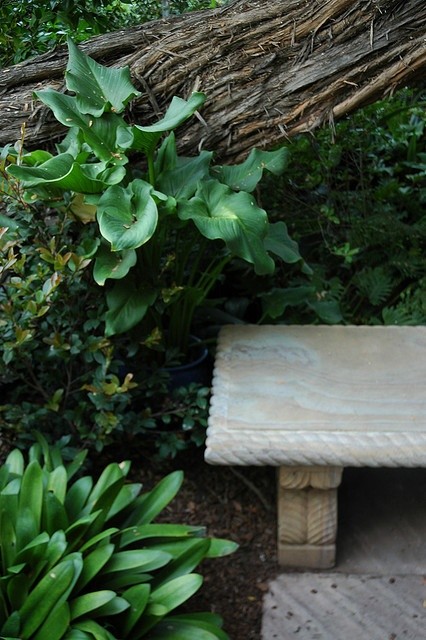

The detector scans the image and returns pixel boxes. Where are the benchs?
[202,322,425,569]
